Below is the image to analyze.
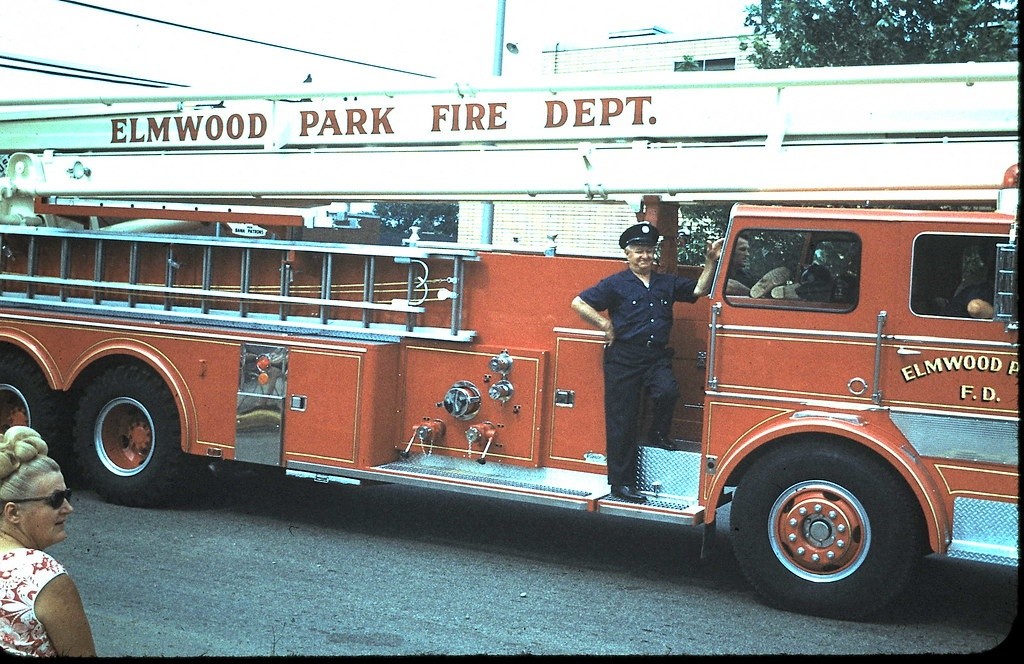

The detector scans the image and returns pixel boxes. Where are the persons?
[0,426,96,657]
[939,280,995,318]
[571,221,725,502]
[728,234,757,289]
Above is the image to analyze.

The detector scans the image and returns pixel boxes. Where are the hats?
[619,224,659,249]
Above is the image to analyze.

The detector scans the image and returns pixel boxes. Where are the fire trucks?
[1,61,1019,621]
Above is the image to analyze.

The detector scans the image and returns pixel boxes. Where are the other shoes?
[771,282,804,301]
[749,266,792,299]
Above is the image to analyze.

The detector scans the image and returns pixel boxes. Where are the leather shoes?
[611,485,646,501]
[649,431,677,450]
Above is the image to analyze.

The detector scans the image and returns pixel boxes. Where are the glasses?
[5,488,71,510]
